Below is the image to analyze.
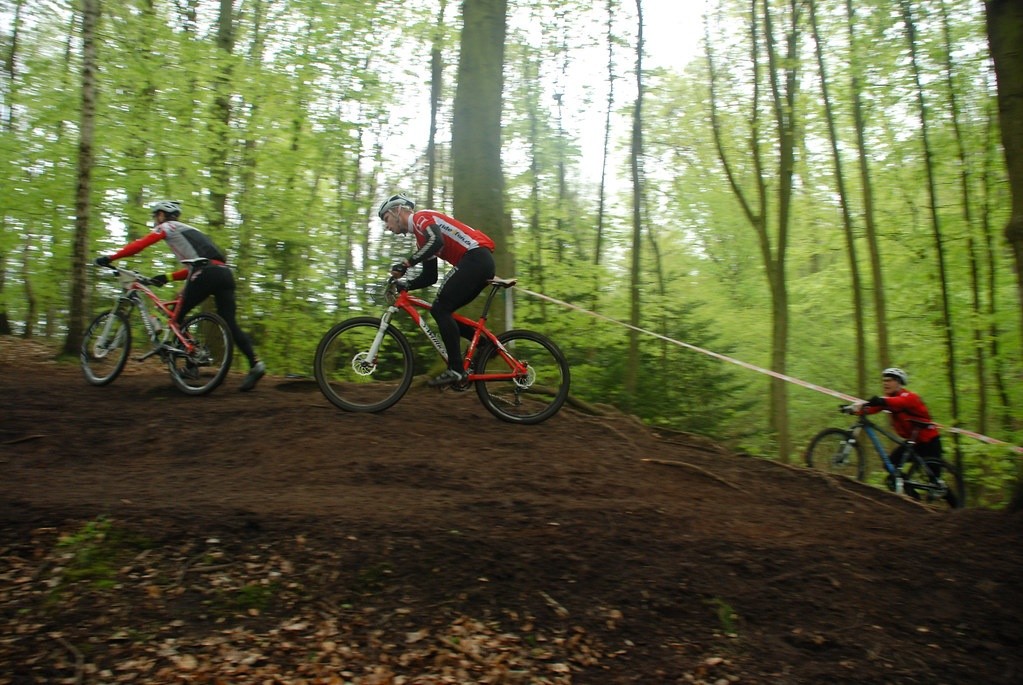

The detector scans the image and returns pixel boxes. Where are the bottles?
[151,314,163,333]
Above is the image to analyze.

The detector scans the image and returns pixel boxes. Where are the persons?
[839,367,956,509]
[93,201,266,391]
[378,193,496,386]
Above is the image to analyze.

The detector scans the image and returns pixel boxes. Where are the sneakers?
[472,348,499,366]
[428,367,468,385]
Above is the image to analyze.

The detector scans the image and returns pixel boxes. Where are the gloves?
[149,275,169,287]
[94,256,112,267]
[867,396,883,407]
[840,404,855,415]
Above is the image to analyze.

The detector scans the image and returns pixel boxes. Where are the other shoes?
[238,361,265,392]
[176,366,200,380]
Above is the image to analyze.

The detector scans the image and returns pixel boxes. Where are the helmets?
[378,193,416,219]
[151,201,182,218]
[882,368,907,385]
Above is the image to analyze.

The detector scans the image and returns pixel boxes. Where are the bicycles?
[803,405,964,513]
[312,267,571,426]
[80,257,235,399]
